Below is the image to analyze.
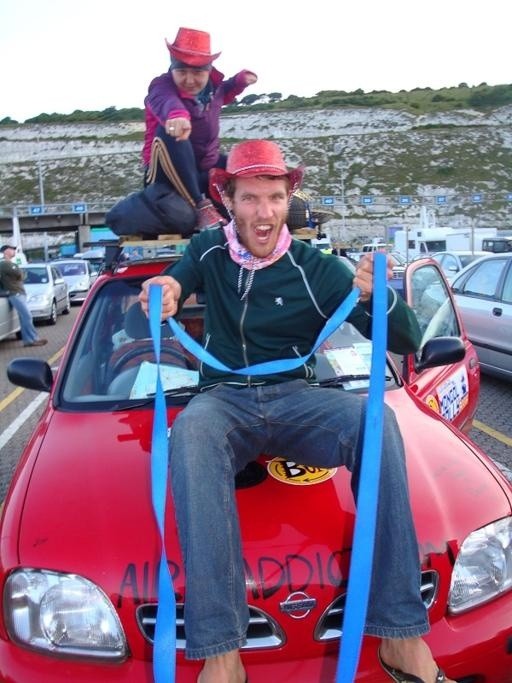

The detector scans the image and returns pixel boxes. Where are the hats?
[1,243,18,254]
[205,138,304,206]
[164,24,223,68]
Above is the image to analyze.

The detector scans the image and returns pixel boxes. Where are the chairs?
[106,291,208,393]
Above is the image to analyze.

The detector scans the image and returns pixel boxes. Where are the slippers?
[376,645,446,683]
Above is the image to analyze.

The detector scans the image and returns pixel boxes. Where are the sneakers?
[195,199,228,232]
[23,338,48,347]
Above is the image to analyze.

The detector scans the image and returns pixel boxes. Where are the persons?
[104,25,314,239]
[136,138,463,682]
[0,240,48,346]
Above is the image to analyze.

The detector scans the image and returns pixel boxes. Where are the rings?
[168,126,175,131]
